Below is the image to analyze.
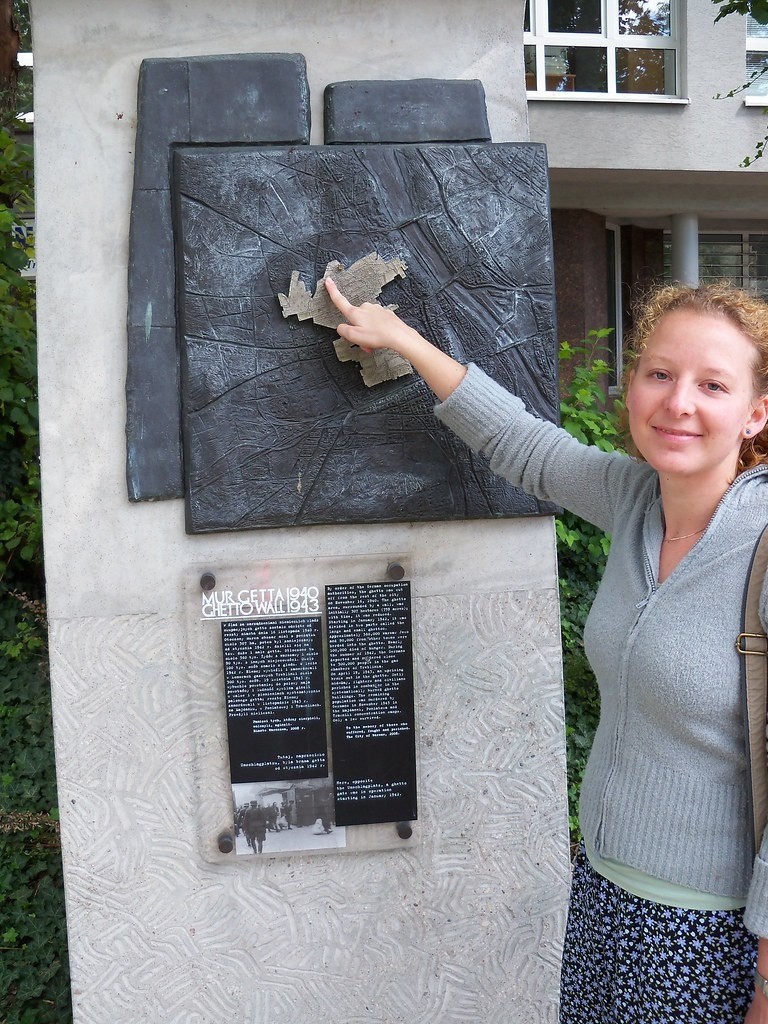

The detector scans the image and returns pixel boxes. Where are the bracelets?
[755,968,768,998]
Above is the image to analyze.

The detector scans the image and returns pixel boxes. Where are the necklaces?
[661,524,708,542]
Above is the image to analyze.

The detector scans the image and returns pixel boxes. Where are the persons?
[323,275,767,1023]
